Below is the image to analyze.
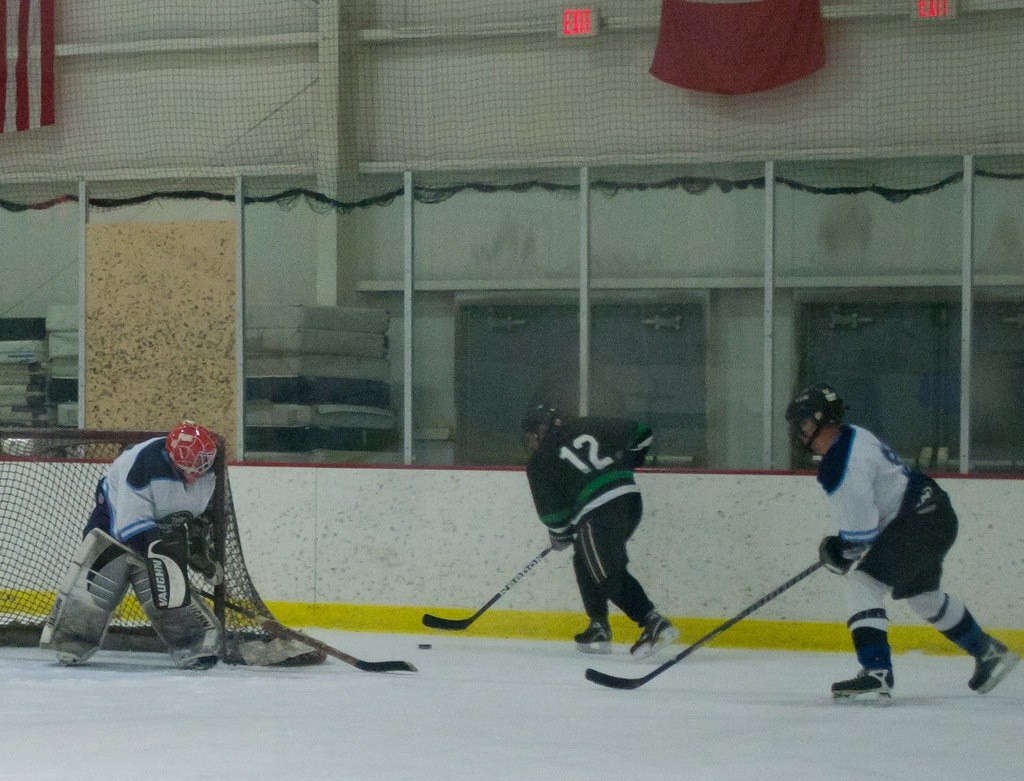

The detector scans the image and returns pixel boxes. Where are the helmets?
[520,402,562,432]
[165,419,217,484]
[786,382,844,426]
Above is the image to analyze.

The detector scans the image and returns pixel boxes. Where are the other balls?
[419,644,431,649]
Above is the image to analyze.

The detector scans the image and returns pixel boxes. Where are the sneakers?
[967,634,1021,695]
[630,611,678,660]
[574,616,614,654]
[831,669,894,707]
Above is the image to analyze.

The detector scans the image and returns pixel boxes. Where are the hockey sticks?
[585,559,825,690]
[125,553,421,675]
[422,545,554,632]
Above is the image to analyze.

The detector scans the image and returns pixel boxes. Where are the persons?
[519,404,677,660]
[40,421,226,668]
[785,383,1020,703]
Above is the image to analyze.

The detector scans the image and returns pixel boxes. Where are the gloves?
[819,536,854,576]
[548,524,577,551]
